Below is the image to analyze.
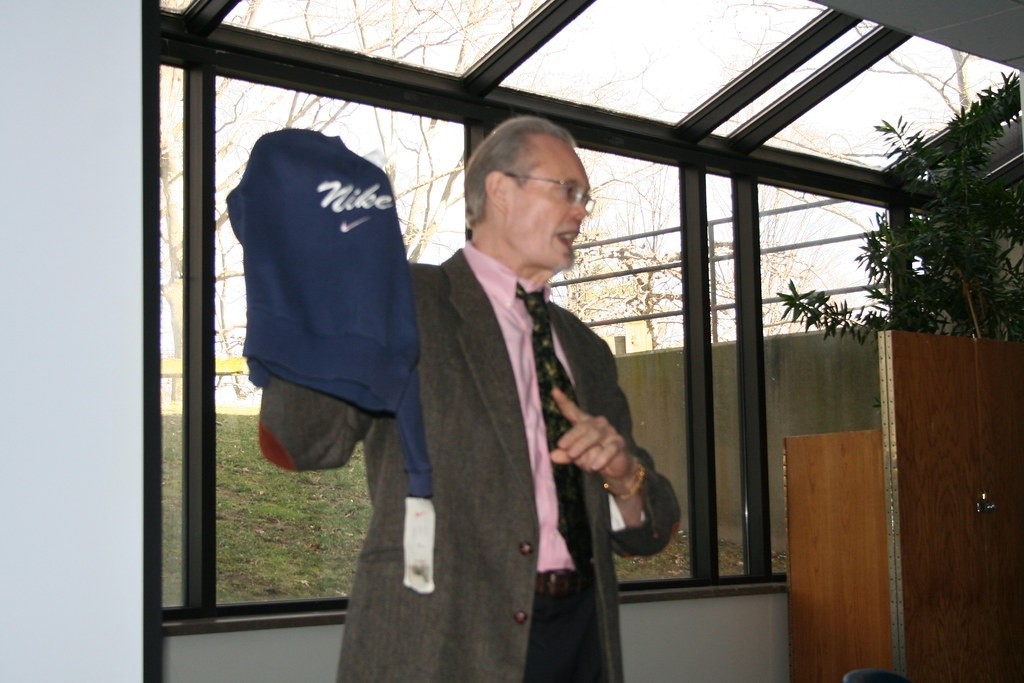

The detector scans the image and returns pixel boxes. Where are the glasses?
[503,171,596,216]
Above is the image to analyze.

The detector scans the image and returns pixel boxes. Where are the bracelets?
[599,460,647,503]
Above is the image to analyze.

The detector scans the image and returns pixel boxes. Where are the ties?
[516,281,599,579]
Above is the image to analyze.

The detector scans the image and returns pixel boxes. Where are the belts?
[532,567,594,600]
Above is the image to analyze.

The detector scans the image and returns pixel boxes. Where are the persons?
[256,112,688,683]
